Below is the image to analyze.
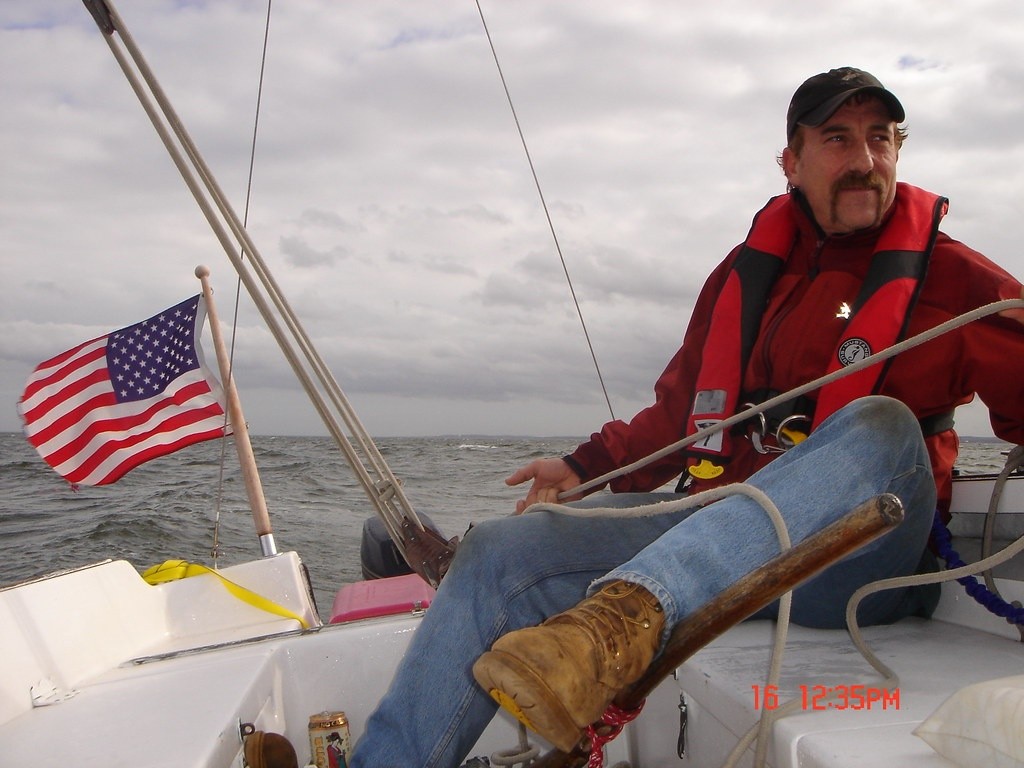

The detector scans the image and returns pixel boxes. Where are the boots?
[472,580,665,754]
[244,731,298,768]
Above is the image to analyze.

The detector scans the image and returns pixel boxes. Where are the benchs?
[605,467,1024,768]
[0,645,284,768]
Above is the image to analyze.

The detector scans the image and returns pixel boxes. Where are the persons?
[343,65,1024,768]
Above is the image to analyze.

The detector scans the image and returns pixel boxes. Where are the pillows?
[909,674,1024,768]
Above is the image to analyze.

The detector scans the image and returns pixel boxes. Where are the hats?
[786,66,905,147]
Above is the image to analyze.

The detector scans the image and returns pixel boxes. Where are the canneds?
[307,710,352,768]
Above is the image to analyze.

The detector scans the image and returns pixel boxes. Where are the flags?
[16,289,245,490]
